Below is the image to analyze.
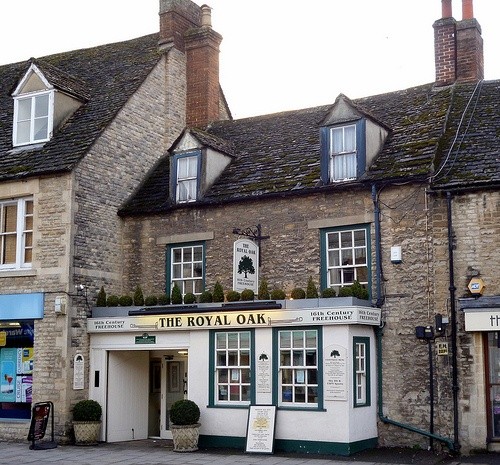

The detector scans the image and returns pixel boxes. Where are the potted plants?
[71,399,103,446]
[168,399,202,453]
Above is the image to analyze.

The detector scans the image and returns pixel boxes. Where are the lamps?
[69,283,85,297]
[232,226,257,239]
